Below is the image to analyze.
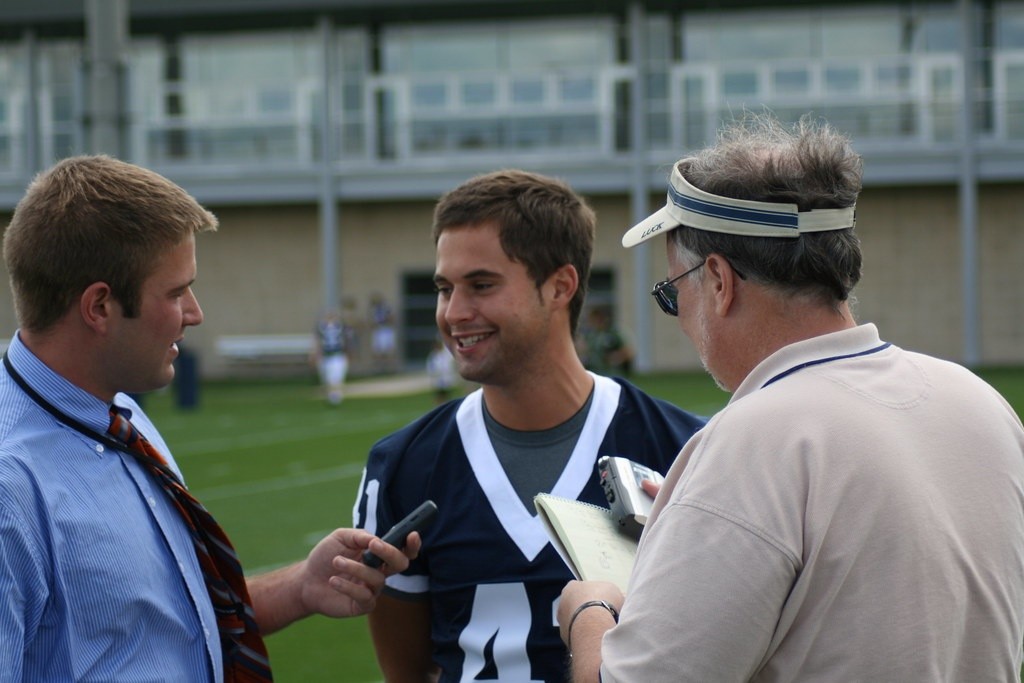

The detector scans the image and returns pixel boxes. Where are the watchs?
[567,600,618,648]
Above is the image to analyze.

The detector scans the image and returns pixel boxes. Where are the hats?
[621,157,856,249]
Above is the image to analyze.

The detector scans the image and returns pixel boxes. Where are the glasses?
[650,254,748,316]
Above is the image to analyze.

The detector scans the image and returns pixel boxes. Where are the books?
[532,489,642,602]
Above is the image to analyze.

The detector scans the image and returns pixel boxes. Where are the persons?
[0,151,423,683]
[314,115,1018,683]
[169,344,196,411]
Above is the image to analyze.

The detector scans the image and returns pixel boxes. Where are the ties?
[106,410,276,683]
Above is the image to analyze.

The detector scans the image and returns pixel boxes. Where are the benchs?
[211,334,318,384]
